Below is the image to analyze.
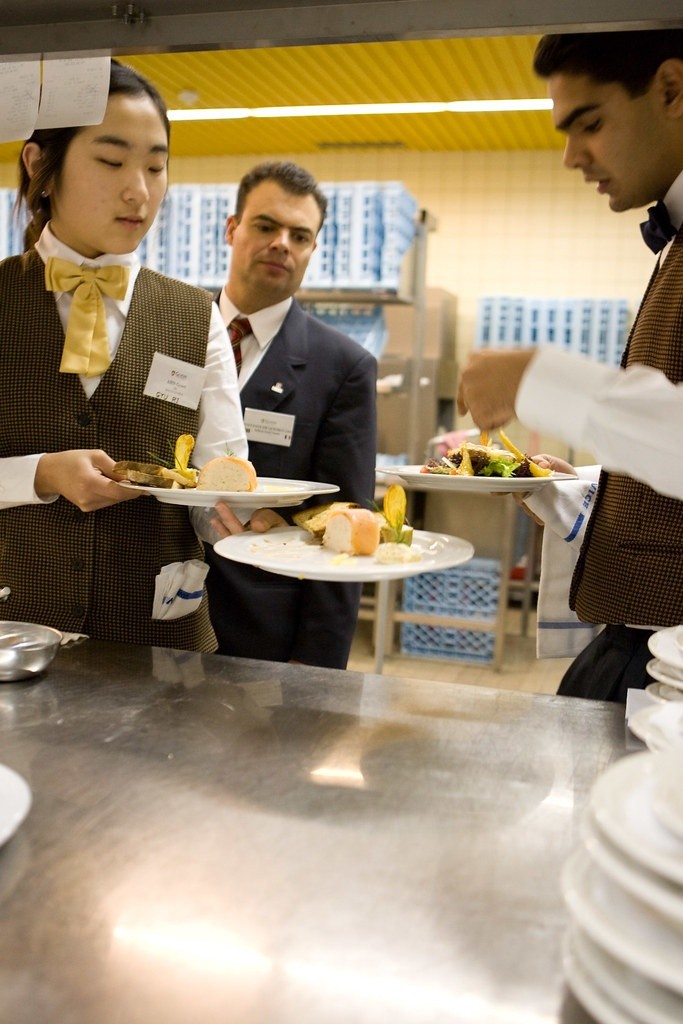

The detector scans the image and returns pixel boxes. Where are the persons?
[456,30,683,704]
[0,60,293,655]
[209,162,380,669]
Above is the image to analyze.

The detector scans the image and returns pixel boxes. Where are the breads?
[291,500,361,537]
[322,508,380,556]
[111,461,198,489]
[197,456,257,493]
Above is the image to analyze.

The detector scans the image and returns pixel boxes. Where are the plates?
[559,624,683,1024]
[118,477,340,507]
[374,464,579,492]
[213,526,475,582]
[1,763,34,851]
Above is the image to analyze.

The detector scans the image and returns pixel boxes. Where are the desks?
[0,636,683,1024]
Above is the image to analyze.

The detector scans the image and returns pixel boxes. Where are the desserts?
[365,483,413,546]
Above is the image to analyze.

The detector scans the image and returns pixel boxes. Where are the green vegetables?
[429,457,521,479]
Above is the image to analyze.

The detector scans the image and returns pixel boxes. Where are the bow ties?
[45,257,130,377]
[639,199,677,254]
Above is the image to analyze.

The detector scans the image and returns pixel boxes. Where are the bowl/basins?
[0,621,64,681]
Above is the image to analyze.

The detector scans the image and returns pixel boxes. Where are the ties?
[228,316,254,380]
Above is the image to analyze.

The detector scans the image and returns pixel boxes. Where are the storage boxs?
[476,296,628,369]
[399,557,502,662]
[0,180,419,359]
